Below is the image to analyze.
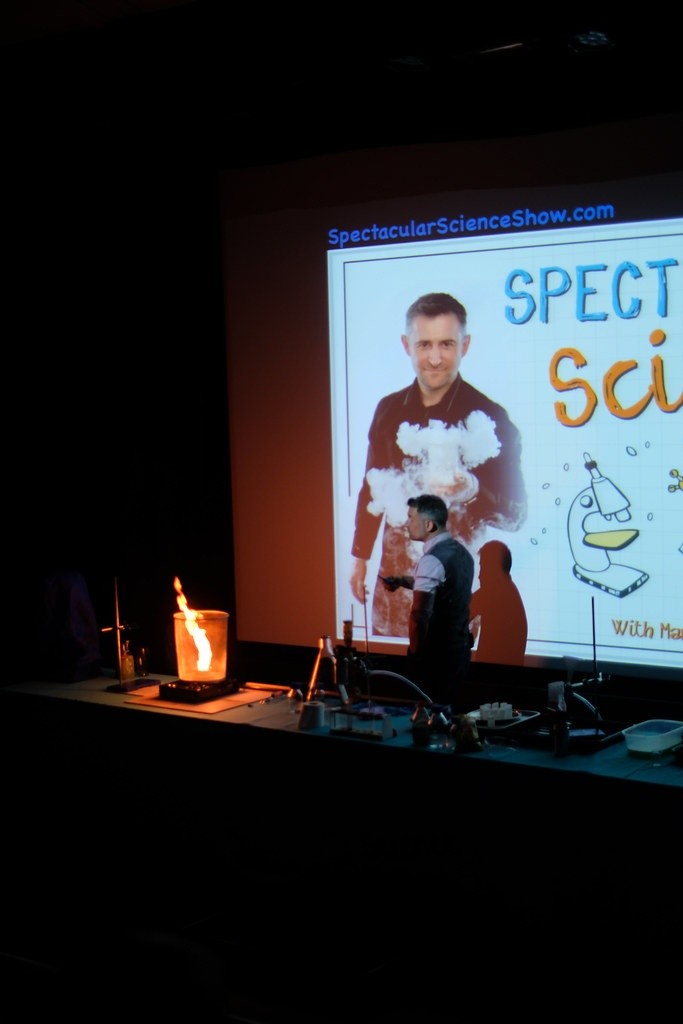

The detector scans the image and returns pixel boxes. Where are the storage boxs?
[621,719,683,753]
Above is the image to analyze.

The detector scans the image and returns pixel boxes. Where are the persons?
[349,293,520,636]
[382,495,477,755]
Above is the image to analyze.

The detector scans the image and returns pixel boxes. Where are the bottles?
[340,621,353,688]
[318,634,337,690]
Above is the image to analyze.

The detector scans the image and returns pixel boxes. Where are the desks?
[247,701,616,892]
[576,744,683,899]
[0,669,307,830]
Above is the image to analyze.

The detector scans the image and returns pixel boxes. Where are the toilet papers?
[298,703,325,730]
